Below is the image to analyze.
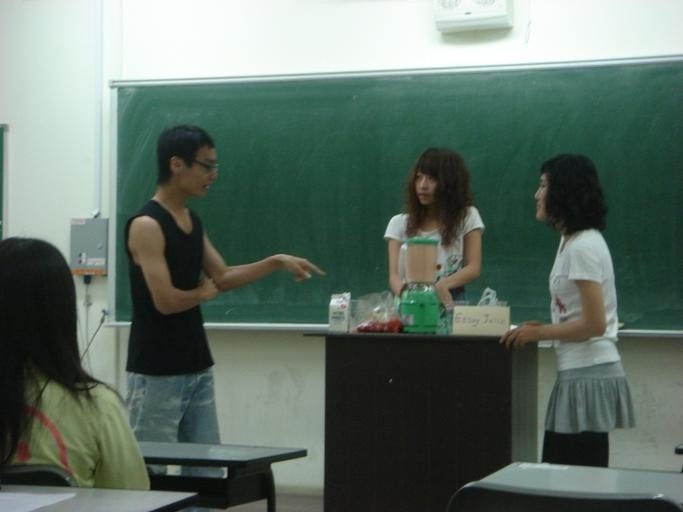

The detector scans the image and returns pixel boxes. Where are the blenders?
[401,238,442,339]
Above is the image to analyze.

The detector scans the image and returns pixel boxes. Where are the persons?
[495,152,640,468]
[125,124,328,476]
[382,145,488,329]
[0,236,154,491]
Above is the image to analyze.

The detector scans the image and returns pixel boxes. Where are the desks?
[447,459,682,512]
[137,441,306,512]
[0,482,200,512]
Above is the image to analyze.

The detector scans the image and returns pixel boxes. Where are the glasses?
[193,160,219,173]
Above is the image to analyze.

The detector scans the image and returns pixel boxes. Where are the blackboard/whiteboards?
[105,54,683,337]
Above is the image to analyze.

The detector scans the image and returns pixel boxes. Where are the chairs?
[1,464,78,487]
[446,480,682,512]
